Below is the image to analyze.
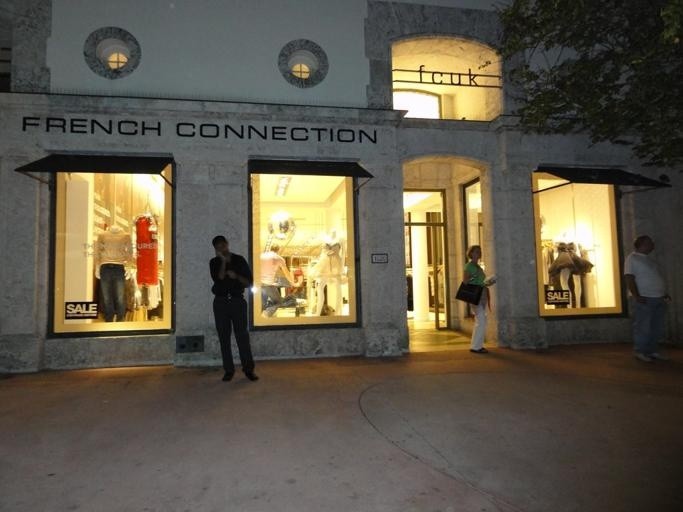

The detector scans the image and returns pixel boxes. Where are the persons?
[134,214,159,287]
[288,258,304,298]
[463,246,496,353]
[308,227,346,317]
[548,232,594,309]
[260,244,294,317]
[210,236,259,381]
[95,224,133,322]
[624,236,673,362]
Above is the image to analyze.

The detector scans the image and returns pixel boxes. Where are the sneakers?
[633,352,671,362]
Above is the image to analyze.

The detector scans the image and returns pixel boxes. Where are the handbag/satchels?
[456,281,481,306]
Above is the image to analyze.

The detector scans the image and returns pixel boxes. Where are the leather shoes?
[222,368,235,382]
[470,347,488,353]
[242,368,259,382]
